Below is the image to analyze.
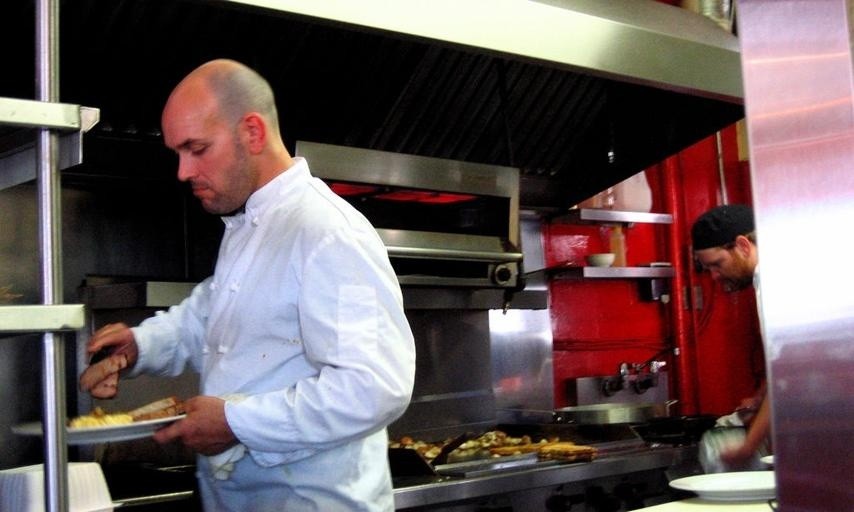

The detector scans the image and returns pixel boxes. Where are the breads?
[129,395,181,422]
[490,443,594,462]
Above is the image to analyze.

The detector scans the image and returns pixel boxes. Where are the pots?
[631,406,754,442]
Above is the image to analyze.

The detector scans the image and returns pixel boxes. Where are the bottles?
[610,222,628,267]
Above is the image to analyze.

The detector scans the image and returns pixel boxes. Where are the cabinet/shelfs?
[544,206,676,303]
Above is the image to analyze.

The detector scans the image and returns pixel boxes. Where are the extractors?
[96,0,742,216]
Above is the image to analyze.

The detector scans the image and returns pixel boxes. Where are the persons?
[86,59,416,512]
[691,204,771,472]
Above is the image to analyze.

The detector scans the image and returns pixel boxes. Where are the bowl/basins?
[586,253,617,268]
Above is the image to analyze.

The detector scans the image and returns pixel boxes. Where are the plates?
[668,455,780,501]
[10,408,184,447]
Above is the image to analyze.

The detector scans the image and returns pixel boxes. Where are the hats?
[691,204,754,251]
